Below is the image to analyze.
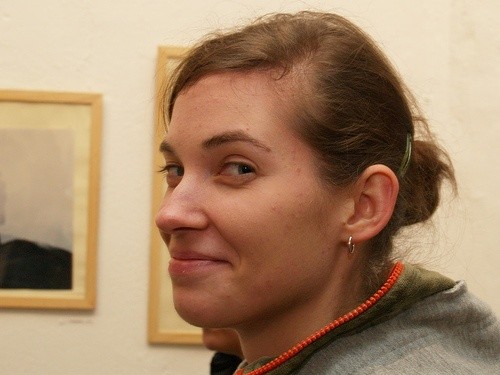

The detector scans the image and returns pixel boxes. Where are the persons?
[202,328,245,375]
[154,11,500,375]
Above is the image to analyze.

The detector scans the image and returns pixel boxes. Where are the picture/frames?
[146,46,207,347]
[0,90,103,311]
